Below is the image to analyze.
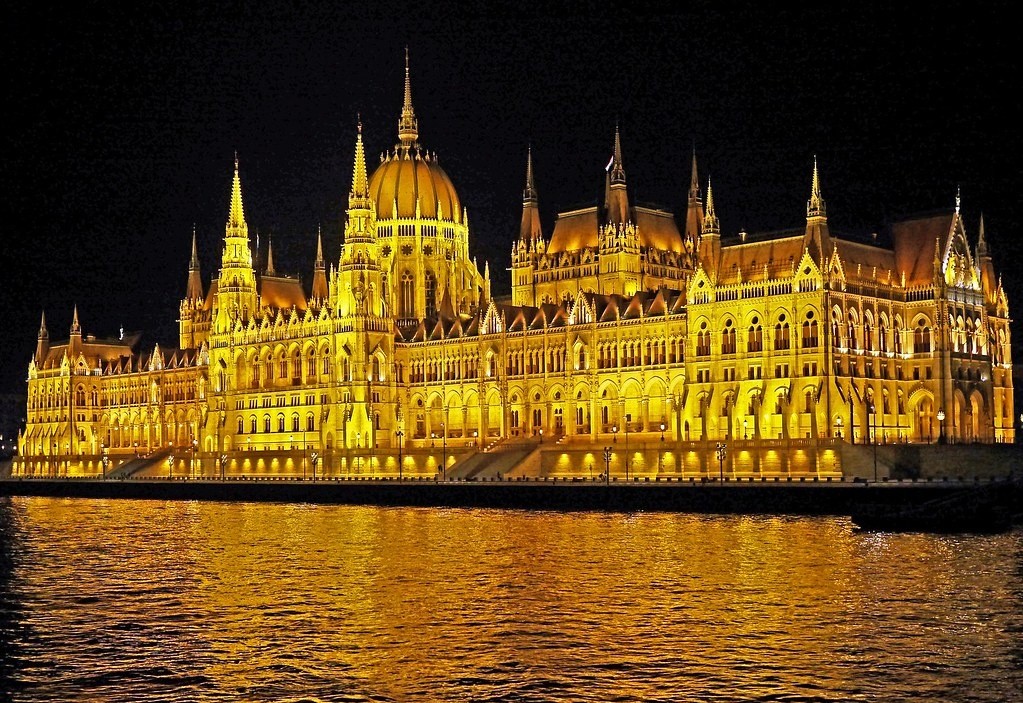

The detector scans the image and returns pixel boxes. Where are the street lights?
[311,453,319,483]
[356,432,361,473]
[192,438,199,480]
[167,455,175,481]
[301,428,306,481]
[716,442,727,485]
[870,405,877,483]
[396,430,403,483]
[603,446,612,485]
[440,423,446,481]
[221,455,227,483]
